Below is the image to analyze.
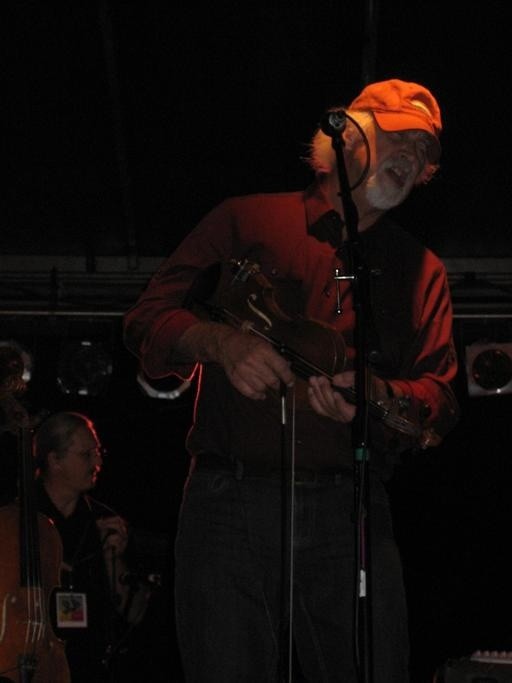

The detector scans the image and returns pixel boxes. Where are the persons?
[29,410,144,682]
[120,75,470,683]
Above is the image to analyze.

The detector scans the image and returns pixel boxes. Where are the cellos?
[1,359,69,682]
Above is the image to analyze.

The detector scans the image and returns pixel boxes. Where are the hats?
[348,79,442,164]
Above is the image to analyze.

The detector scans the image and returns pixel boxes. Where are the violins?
[186,262,433,450]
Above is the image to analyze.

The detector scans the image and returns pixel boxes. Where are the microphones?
[319,109,346,137]
